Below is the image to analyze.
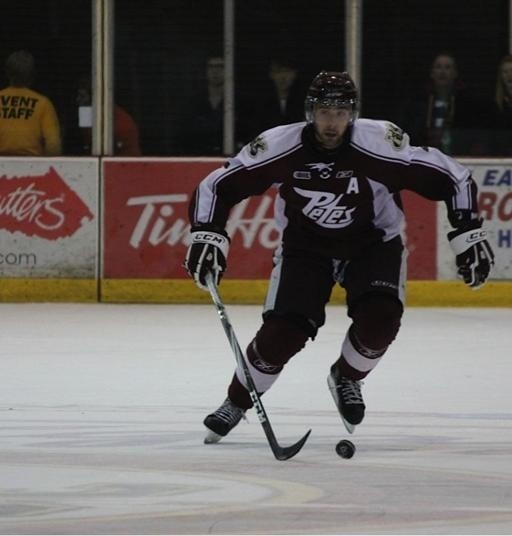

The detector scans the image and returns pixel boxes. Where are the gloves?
[447,216,495,288]
[182,224,231,288]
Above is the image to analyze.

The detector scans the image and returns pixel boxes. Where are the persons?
[185,71,494,437]
[494,57,512,116]
[0,51,62,155]
[179,50,257,158]
[75,84,141,156]
[408,54,478,154]
[254,53,306,131]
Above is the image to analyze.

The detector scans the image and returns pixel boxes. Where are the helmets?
[303,71,360,125]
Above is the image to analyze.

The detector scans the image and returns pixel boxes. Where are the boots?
[330,354,372,425]
[203,372,265,437]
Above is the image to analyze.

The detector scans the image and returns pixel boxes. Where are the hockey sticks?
[203,263,312,460]
